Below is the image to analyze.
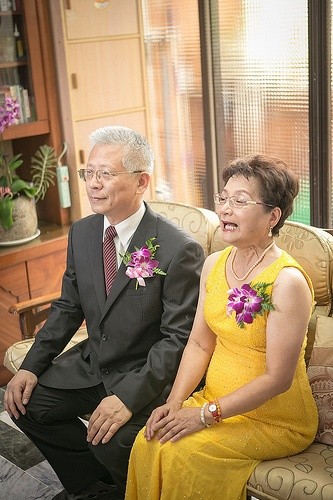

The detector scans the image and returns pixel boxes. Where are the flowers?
[118,237,167,289]
[225,280,276,328]
[0,93,57,229]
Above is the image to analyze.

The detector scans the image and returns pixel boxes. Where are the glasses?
[77,169,142,182]
[212,193,275,208]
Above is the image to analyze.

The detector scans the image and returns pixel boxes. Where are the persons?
[3,126,205,500]
[124,153,319,500]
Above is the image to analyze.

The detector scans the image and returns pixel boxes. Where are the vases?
[0,195,41,247]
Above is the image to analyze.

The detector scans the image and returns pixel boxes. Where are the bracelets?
[200,398,222,428]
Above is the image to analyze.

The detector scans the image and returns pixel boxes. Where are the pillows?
[304,314,333,446]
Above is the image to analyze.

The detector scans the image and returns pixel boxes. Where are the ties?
[102,226,117,297]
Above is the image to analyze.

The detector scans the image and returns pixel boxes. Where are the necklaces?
[230,240,276,281]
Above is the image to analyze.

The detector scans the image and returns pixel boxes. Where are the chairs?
[3,201,333,500]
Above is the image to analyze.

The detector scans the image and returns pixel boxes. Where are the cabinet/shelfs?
[0,0,75,390]
